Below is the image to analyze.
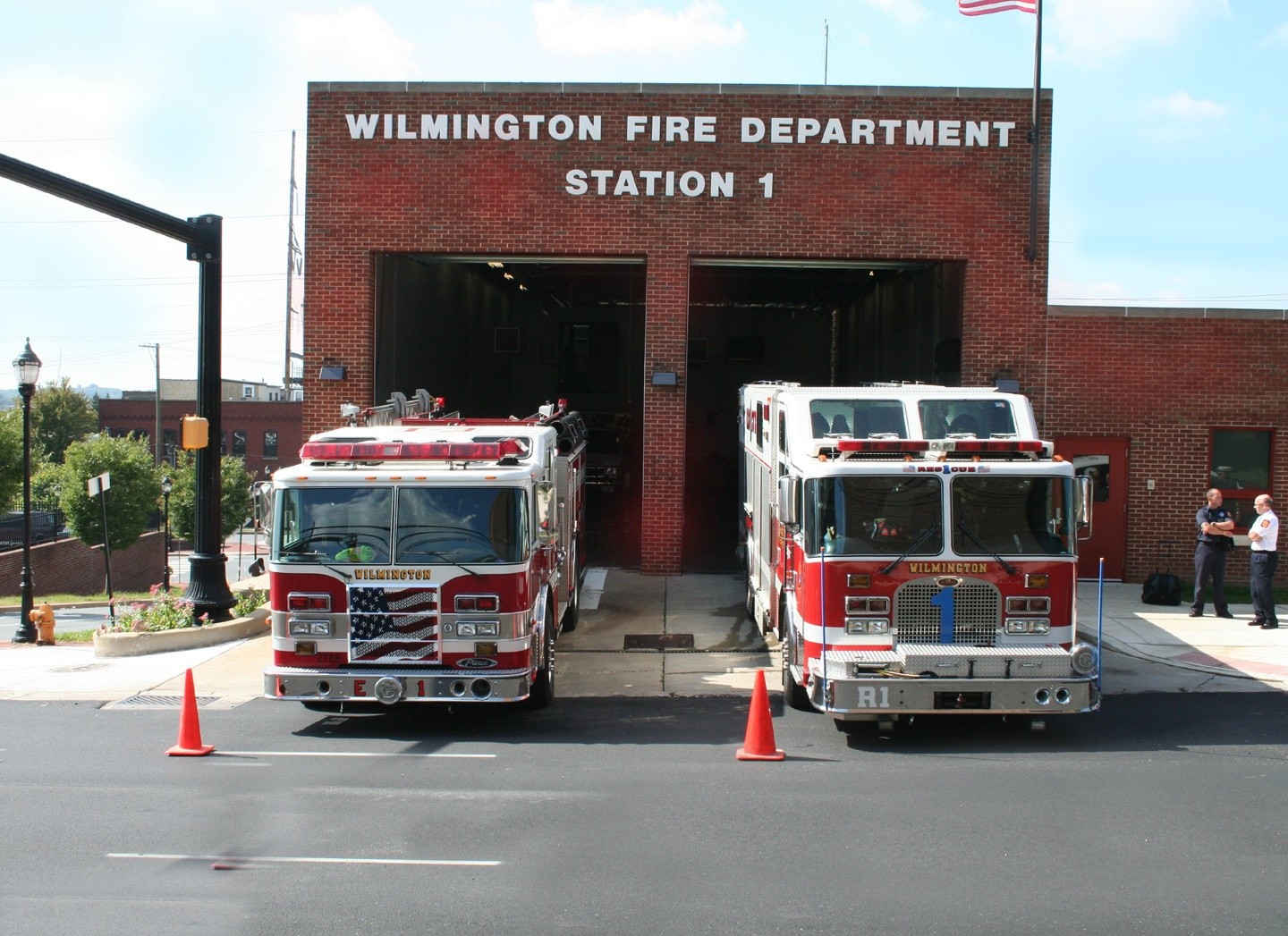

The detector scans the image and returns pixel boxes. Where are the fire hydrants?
[29,601,56,645]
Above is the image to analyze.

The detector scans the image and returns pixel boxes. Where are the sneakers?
[1248,616,1266,626]
[1189,611,1203,616]
[1261,619,1279,629]
[1218,612,1233,618]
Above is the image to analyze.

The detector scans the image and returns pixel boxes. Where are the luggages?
[1142,541,1182,607]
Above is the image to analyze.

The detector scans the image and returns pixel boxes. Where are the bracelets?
[1211,522,1216,526]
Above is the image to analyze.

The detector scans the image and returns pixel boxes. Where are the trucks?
[575,411,632,501]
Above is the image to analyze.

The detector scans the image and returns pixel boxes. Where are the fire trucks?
[736,378,1107,731]
[248,388,591,711]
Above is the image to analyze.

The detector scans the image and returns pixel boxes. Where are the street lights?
[11,335,43,644]
[161,477,172,592]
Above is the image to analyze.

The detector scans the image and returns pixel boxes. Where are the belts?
[1199,541,1222,546]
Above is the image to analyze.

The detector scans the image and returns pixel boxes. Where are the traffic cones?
[163,668,215,757]
[736,670,787,761]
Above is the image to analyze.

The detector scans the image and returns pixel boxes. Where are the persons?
[1247,495,1280,630]
[1188,489,1235,619]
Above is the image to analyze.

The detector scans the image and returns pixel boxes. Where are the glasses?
[1255,503,1262,506]
